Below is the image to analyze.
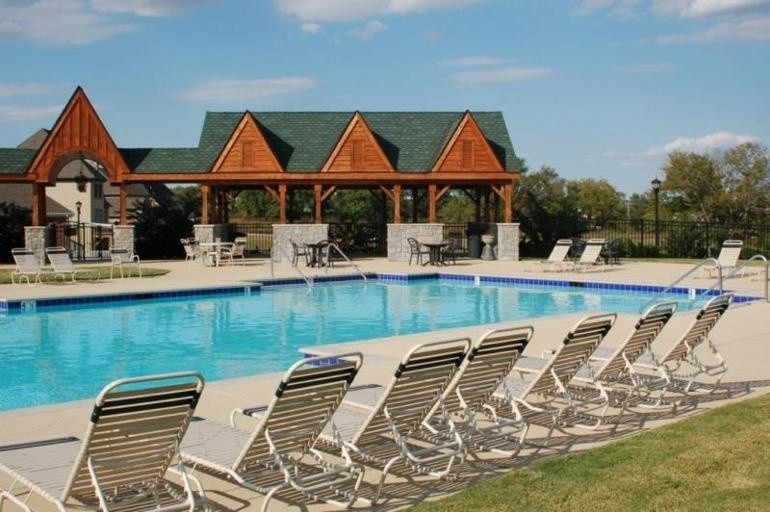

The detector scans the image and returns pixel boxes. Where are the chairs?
[694,238,743,279]
[227,335,475,503]
[105,346,365,512]
[535,237,606,273]
[10,246,142,286]
[180,236,246,267]
[407,236,458,268]
[2,368,215,512]
[288,236,347,267]
[313,292,732,487]
